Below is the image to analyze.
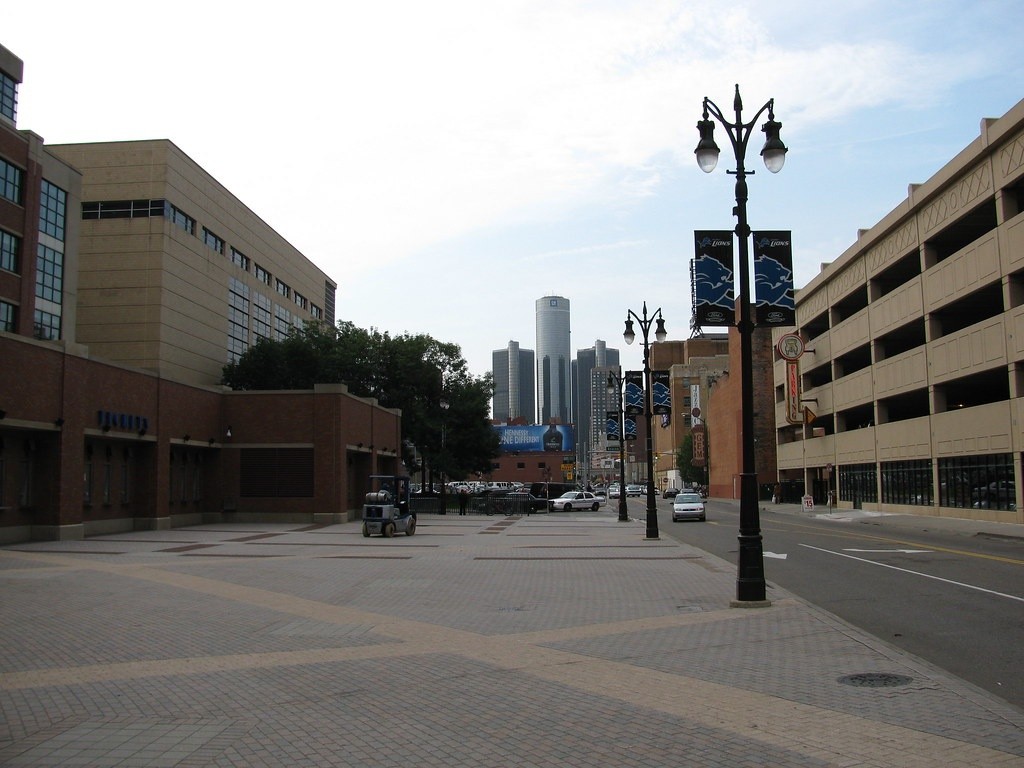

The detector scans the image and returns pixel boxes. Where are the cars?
[680,489,695,494]
[548,492,607,511]
[642,486,660,495]
[670,493,707,522]
[608,485,628,499]
[663,488,679,499]
[972,480,1016,502]
[627,485,641,497]
[410,481,584,514]
[595,488,607,496]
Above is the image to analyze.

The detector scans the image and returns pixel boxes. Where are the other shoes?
[459,514,462,515]
[463,514,466,515]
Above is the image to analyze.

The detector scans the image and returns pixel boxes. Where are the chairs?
[366,492,399,519]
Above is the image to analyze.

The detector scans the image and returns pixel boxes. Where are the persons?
[457,489,468,516]
[378,482,392,501]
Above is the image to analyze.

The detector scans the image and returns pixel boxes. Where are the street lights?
[605,365,629,522]
[694,84,788,608]
[623,301,668,540]
[681,413,707,499]
[439,395,450,515]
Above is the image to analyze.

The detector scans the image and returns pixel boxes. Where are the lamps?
[183,434,190,441]
[368,445,375,449]
[382,447,388,451]
[101,425,112,433]
[207,437,215,444]
[55,416,66,426]
[357,442,364,448]
[0,409,7,419]
[138,428,146,435]
[226,428,232,436]
[391,449,396,453]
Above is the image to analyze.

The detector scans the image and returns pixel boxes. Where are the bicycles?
[486,499,514,516]
